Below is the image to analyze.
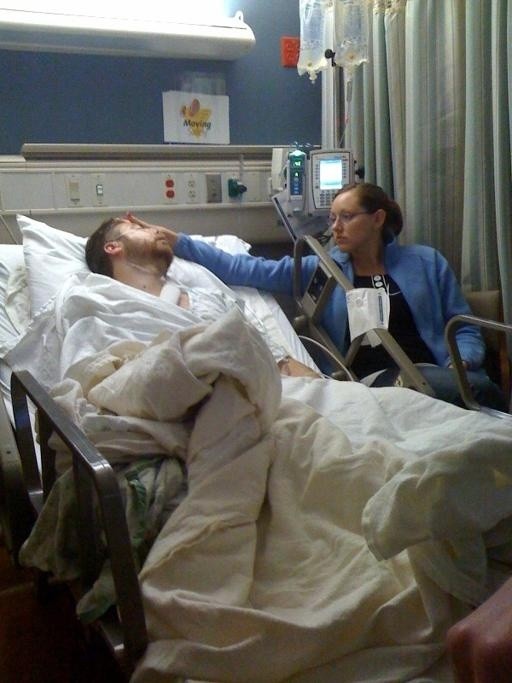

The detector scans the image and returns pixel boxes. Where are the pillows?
[14,212,258,329]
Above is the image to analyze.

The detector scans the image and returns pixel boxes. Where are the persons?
[123,182,507,410]
[57,218,322,396]
[445,571,512,683]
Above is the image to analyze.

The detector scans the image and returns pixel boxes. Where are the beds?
[0,231,512,682]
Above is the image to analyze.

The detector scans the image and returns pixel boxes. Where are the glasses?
[327,210,367,226]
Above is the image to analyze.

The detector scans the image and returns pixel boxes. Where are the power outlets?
[183,172,201,204]
